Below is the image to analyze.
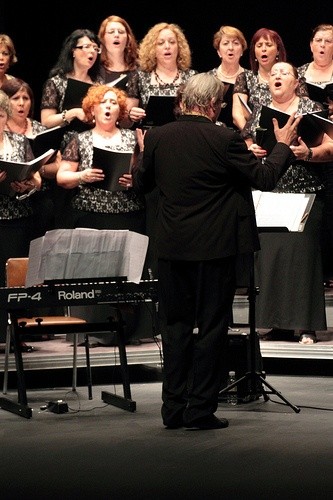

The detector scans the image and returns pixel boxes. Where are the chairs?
[2,255,86,395]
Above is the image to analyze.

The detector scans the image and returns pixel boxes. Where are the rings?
[126,183,131,187]
[259,150,262,154]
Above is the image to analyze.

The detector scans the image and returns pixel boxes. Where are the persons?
[205,26,287,292]
[117,22,199,135]
[92,15,138,98]
[55,86,161,345]
[242,61,333,343]
[0,89,42,353]
[0,33,62,341]
[41,28,102,155]
[295,25,333,288]
[132,72,303,430]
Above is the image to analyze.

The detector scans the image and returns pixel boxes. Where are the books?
[61,74,128,110]
[90,146,133,193]
[260,104,328,147]
[144,96,180,125]
[1,149,55,187]
[28,126,62,156]
[305,80,333,101]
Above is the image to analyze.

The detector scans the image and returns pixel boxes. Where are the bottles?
[227,372,238,406]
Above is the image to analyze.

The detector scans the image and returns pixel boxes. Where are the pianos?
[0,275,161,419]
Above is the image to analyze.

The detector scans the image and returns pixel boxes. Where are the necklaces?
[153,67,181,94]
[257,69,269,83]
[109,61,127,72]
[218,64,240,78]
[6,118,28,135]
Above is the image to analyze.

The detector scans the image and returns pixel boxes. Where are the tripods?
[215,194,317,412]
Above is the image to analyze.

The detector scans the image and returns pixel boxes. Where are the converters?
[48,401,68,413]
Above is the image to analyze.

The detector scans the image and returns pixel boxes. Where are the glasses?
[218,99,227,108]
[74,45,102,53]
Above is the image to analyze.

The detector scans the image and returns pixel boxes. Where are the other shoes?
[299,333,315,344]
[261,329,295,340]
[184,418,229,430]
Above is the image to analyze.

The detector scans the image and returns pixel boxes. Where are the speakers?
[67,300,157,347]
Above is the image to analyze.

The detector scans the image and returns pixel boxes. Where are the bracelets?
[62,109,71,126]
[78,171,86,189]
[304,148,313,162]
[128,109,134,123]
[42,164,46,178]
